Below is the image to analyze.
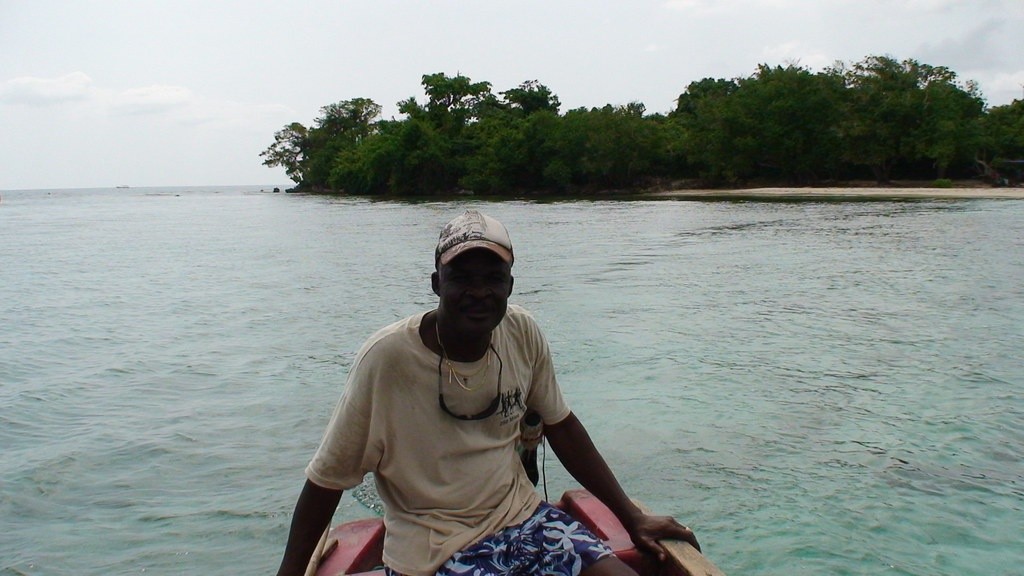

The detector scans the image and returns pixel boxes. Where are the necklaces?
[433,322,492,392]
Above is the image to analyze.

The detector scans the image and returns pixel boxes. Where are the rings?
[686,526,692,532]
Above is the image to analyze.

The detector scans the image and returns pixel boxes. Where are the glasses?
[439,344,502,420]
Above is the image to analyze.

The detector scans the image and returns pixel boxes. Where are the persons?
[277,210,703,576]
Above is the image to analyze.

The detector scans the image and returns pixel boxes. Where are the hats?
[435,209,515,269]
